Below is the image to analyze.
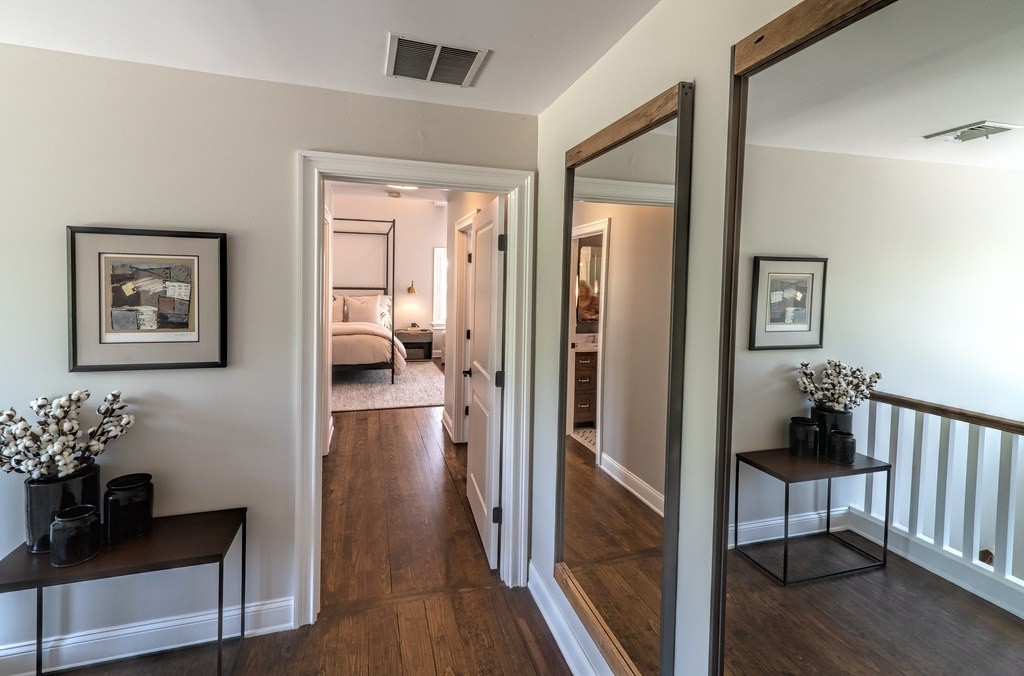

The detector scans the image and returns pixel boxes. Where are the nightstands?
[395,329,434,360]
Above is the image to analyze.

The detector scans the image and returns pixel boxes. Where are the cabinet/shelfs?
[573,352,597,429]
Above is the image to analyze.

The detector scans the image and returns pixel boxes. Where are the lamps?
[408,280,416,293]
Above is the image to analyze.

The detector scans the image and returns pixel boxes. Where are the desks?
[0,506,248,676]
[735,449,893,587]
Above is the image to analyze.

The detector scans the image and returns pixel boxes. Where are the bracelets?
[592,315,596,320]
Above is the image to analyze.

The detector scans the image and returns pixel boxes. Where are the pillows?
[331,295,393,328]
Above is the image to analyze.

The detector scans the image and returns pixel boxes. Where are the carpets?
[329,360,446,412]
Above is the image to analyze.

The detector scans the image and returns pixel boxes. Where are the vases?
[810,406,853,456]
[24,457,101,554]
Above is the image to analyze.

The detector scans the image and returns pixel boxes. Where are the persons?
[577,279,600,321]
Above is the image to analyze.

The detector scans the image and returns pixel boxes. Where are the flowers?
[797,358,883,413]
[0,387,135,482]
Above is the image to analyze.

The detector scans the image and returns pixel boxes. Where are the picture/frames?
[748,256,829,351]
[65,224,228,372]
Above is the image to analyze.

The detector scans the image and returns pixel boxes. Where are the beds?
[330,218,396,382]
